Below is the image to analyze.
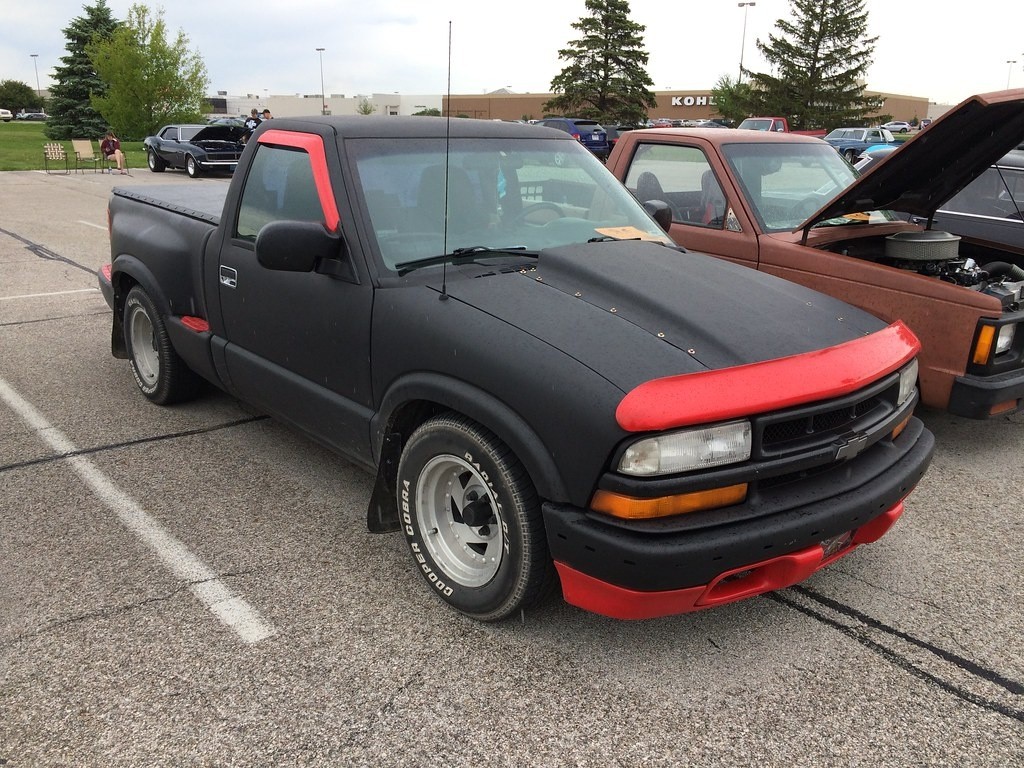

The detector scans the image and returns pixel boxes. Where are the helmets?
[251,109,258,114]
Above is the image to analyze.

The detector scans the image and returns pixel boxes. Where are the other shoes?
[121,172,126,175]
[117,167,121,169]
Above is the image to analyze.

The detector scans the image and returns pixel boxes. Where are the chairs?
[71,138,103,175]
[98,139,130,176]
[43,142,72,175]
[282,159,323,222]
[636,169,729,223]
[417,165,478,239]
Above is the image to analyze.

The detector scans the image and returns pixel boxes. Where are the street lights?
[316,47,326,113]
[1005,59,1016,93]
[30,53,40,96]
[738,2,757,83]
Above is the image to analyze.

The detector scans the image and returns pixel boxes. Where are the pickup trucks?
[95,113,936,626]
[500,87,1024,424]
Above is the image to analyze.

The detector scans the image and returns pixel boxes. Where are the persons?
[100,131,127,175]
[263,109,274,119]
[242,109,262,143]
[735,156,782,195]
[464,151,523,217]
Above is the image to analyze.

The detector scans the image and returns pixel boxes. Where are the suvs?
[600,126,636,152]
[532,117,610,161]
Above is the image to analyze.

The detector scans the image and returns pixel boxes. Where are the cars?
[919,118,932,130]
[143,123,252,178]
[635,118,730,129]
[0,109,14,122]
[491,118,540,125]
[204,115,247,126]
[813,149,1024,268]
[812,126,907,198]
[875,121,912,134]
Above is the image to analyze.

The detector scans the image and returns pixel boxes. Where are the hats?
[260,109,270,114]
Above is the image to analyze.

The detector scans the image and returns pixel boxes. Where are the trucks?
[734,117,828,141]
